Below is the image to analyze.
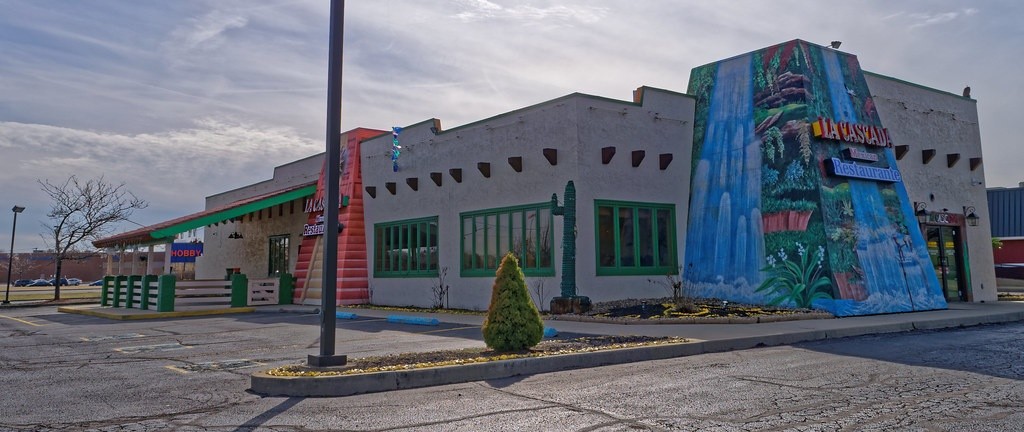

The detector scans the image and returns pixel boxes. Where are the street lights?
[3,205,25,303]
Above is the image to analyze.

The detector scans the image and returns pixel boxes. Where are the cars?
[89,280,104,286]
[13,277,82,287]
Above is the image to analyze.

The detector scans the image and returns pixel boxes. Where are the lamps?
[826,41,842,49]
[228,221,243,239]
[963,206,980,226]
[914,201,931,224]
